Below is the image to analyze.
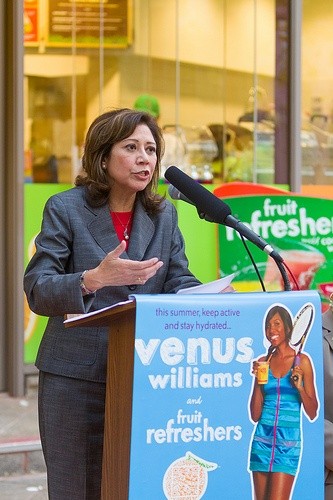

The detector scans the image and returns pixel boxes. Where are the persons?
[248,306,319,500]
[133,95,189,171]
[23,108,238,500]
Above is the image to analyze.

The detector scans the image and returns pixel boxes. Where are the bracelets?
[79,269,97,295]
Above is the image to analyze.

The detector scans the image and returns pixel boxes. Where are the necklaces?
[106,199,134,239]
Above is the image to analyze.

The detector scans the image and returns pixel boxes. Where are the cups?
[257,362,270,383]
[265,239,324,291]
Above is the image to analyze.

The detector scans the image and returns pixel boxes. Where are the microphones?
[165,166,293,293]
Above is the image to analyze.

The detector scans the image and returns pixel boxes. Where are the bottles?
[190,166,200,183]
[201,165,214,184]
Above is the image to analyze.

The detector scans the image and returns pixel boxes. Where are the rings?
[138,276,141,281]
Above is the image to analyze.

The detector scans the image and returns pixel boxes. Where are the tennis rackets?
[287,302,315,380]
